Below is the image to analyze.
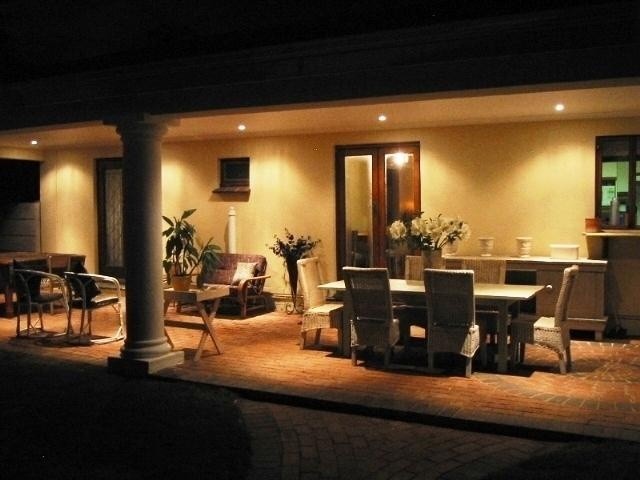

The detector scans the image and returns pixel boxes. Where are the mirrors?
[595,135,640,230]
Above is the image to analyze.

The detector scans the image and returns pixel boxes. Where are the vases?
[422,250,441,270]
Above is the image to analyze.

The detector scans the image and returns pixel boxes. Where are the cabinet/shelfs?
[506,256,609,342]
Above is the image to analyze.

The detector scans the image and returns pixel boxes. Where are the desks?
[163,284,231,361]
[0,252,76,318]
[316,278,545,374]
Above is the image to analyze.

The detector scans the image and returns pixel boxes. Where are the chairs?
[510,265,580,374]
[462,257,507,344]
[342,266,402,369]
[12,269,69,341]
[64,271,125,345]
[296,256,344,354]
[404,255,445,281]
[52,254,86,302]
[12,256,52,314]
[423,268,487,378]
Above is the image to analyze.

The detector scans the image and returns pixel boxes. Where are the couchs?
[197,252,271,319]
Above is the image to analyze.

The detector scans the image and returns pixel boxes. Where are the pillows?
[231,261,258,285]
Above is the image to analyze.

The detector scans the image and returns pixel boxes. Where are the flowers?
[266,228,320,299]
[390,214,470,251]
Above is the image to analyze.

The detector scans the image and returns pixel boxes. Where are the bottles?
[609,197,621,226]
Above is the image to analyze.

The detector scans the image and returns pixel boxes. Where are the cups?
[584,217,601,234]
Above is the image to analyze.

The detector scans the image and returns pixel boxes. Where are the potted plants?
[163,238,182,285]
[161,208,222,291]
